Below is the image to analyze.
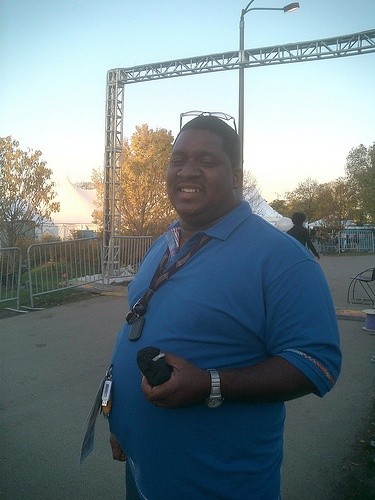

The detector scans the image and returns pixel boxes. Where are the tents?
[305,209,375,252]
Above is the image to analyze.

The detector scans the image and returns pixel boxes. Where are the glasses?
[180,111,237,134]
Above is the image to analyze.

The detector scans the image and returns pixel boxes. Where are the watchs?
[206,369,224,409]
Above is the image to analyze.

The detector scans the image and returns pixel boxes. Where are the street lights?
[238,1,300,218]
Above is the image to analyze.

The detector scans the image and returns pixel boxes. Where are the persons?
[100,115,342,500]
[287,212,320,260]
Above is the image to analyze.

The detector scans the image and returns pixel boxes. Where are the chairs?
[347,267,375,305]
